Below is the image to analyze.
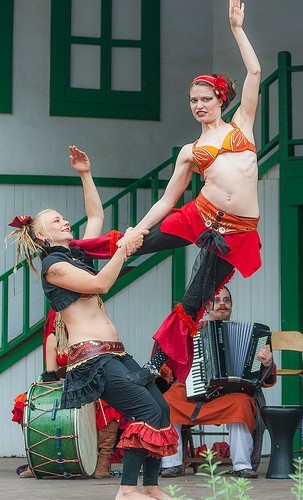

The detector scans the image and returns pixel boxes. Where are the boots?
[93,419,119,479]
[20,470,35,478]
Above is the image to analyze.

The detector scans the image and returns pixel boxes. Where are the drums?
[22,378,98,480]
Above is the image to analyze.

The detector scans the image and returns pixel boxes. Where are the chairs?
[270,331,303,378]
[181,425,233,476]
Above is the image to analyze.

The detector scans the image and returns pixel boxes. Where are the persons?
[162,286,277,478]
[6,145,180,500]
[44,309,119,478]
[70,0,263,393]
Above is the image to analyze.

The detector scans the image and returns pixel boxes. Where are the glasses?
[213,296,231,303]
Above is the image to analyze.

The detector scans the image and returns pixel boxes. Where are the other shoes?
[233,468,258,478]
[115,486,157,500]
[126,364,159,386]
[161,464,183,477]
[141,486,172,500]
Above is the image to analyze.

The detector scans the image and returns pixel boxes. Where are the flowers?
[213,75,229,95]
